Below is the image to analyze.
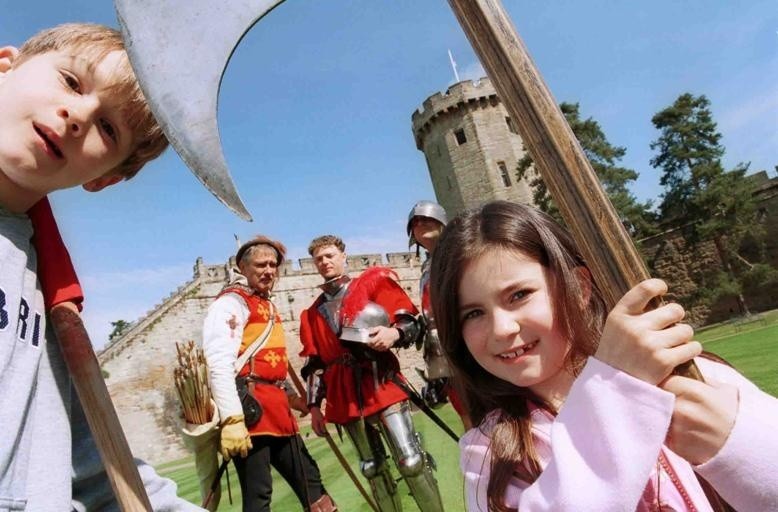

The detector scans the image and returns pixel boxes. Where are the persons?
[0,20,218,512]
[202,234,341,512]
[404,198,475,434]
[427,200,778,511]
[298,235,444,511]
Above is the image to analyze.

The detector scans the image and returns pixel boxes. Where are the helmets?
[340,300,390,344]
[407,201,447,251]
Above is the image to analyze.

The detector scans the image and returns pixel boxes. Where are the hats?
[235,235,286,267]
